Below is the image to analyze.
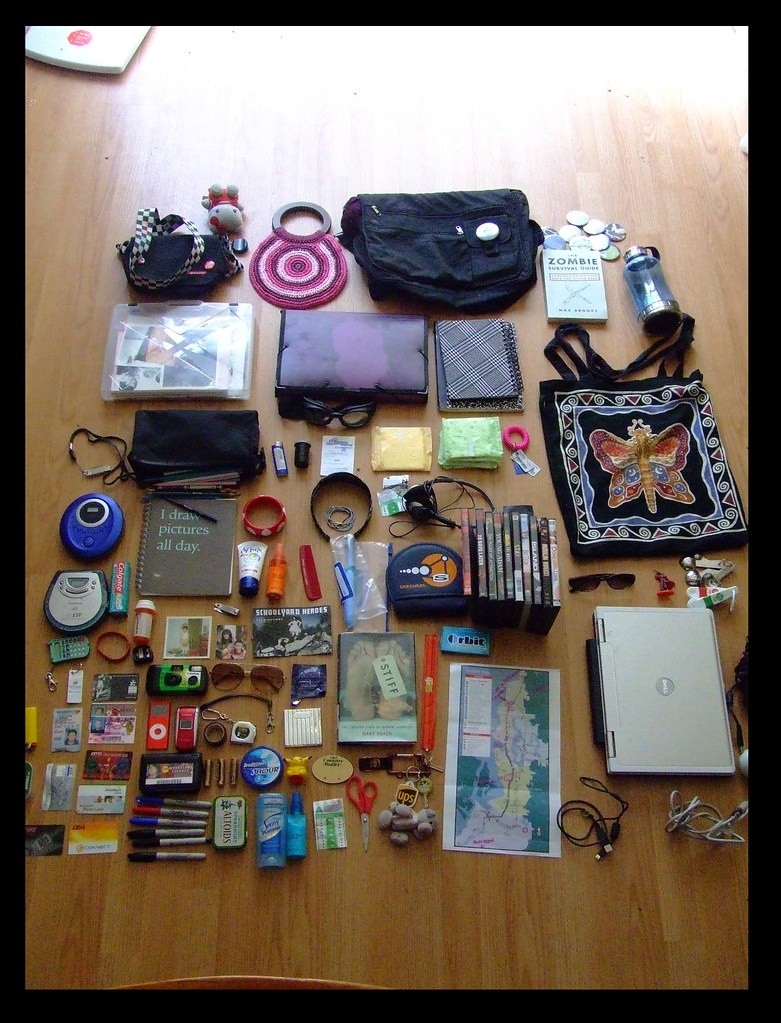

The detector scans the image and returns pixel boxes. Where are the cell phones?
[174,706,199,752]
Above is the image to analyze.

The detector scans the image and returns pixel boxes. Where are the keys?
[417,778,433,808]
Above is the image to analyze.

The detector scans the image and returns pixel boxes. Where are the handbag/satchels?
[339,188,545,315]
[539,312,748,557]
[114,206,247,296]
[247,202,348,309]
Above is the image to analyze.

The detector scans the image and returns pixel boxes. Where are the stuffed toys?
[378,802,436,845]
[201,184,244,233]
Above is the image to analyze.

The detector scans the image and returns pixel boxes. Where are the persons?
[286,616,302,640]
[217,629,246,660]
[94,707,104,716]
[146,764,160,779]
[97,796,124,803]
[179,624,190,655]
[340,640,410,721]
[91,719,104,732]
[63,730,79,745]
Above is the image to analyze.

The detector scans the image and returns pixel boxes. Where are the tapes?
[203,720,228,747]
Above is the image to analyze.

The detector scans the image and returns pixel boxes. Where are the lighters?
[271,441,289,477]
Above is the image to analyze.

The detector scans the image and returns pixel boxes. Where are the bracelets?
[243,495,286,538]
[96,631,131,661]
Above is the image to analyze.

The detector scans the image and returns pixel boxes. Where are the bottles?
[286,791,307,859]
[266,542,287,598]
[622,245,682,337]
[133,599,156,646]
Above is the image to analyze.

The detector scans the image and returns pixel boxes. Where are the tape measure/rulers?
[230,721,257,745]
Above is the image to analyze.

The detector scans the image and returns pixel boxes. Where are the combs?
[299,544,322,600]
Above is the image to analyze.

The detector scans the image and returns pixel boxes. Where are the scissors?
[344,775,379,854]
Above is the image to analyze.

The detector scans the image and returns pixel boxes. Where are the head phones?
[403,475,496,529]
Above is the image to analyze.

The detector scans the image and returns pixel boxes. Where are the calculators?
[48,635,91,664]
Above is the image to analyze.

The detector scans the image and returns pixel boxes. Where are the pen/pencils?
[142,469,240,523]
[126,795,213,863]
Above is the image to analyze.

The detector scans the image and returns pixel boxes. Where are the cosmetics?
[240,746,284,789]
[267,543,287,598]
[212,795,248,850]
[255,793,288,869]
[285,791,307,859]
[238,541,268,598]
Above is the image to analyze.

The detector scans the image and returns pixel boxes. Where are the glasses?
[569,573,635,593]
[300,394,376,428]
[209,662,285,693]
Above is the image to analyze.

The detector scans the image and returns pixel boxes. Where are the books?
[460,508,561,637]
[541,248,609,323]
[337,632,418,747]
[433,319,526,413]
[135,498,237,598]
